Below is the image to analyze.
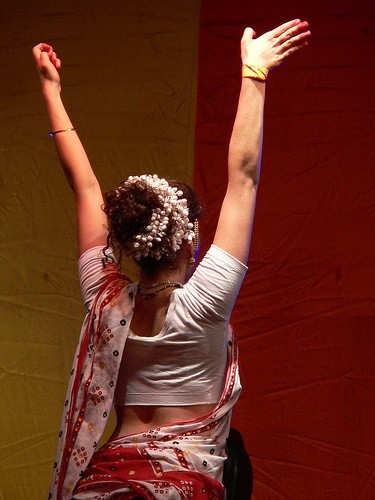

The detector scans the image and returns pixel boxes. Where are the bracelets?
[240,63,269,80]
[48,127,77,137]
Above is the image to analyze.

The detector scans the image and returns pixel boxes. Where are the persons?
[31,18,311,500]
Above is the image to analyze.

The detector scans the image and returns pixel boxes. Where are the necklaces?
[136,280,183,298]
